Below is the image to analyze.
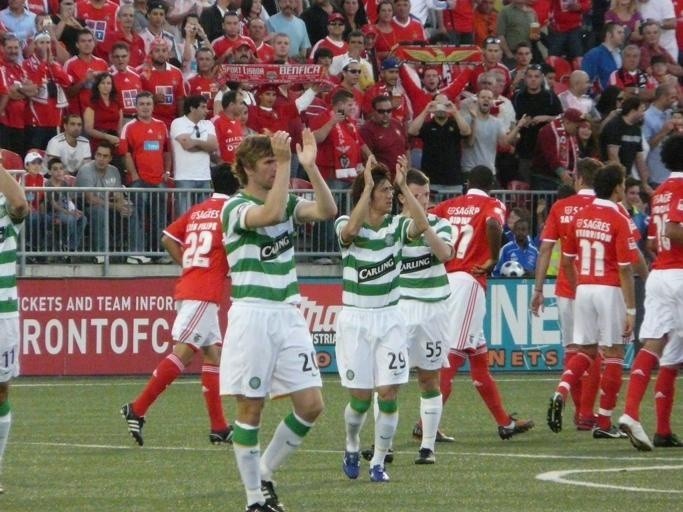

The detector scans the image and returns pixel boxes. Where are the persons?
[529,133,683,450]
[218,127,338,512]
[1,0,681,279]
[0,164,24,482]
[120,153,530,481]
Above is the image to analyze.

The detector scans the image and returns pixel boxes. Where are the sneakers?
[261,480,285,512]
[413,425,456,442]
[210,425,233,446]
[414,448,437,463]
[363,445,394,463]
[25,255,173,264]
[368,461,389,482]
[120,403,144,446]
[498,416,534,439]
[574,412,683,450]
[246,501,283,512]
[547,392,564,434]
[343,448,360,479]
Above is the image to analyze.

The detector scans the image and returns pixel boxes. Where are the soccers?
[500,261,524,277]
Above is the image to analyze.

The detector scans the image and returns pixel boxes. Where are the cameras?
[434,104,448,111]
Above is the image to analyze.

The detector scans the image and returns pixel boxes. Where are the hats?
[566,108,588,128]
[361,24,378,39]
[233,40,251,51]
[340,58,358,74]
[145,1,167,20]
[327,13,344,23]
[24,152,44,169]
[381,58,399,69]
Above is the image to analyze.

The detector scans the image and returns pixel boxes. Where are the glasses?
[346,70,362,74]
[487,38,501,43]
[528,64,542,70]
[376,109,393,113]
[195,126,200,138]
